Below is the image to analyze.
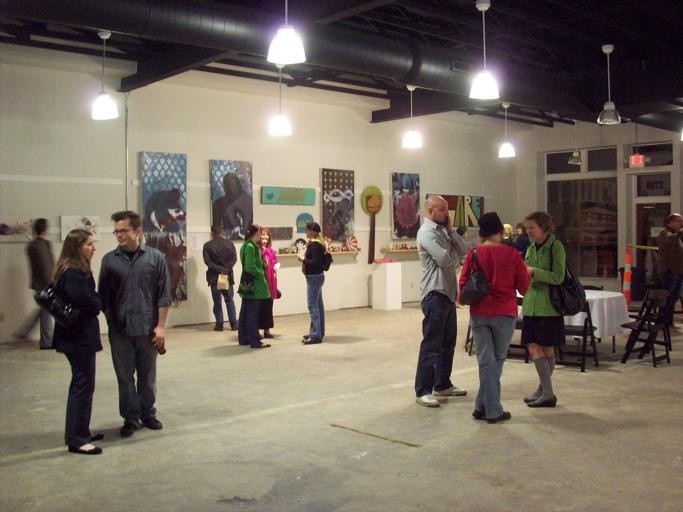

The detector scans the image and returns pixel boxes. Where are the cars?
[555,226,617,249]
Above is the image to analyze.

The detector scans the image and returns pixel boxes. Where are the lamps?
[567,119,585,165]
[596,44,622,125]
[90,31,120,121]
[266,0,307,66]
[468,0,501,100]
[400,84,425,150]
[264,63,295,138]
[497,101,516,158]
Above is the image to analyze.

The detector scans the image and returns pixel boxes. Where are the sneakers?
[258,331,274,346]
[302,334,323,344]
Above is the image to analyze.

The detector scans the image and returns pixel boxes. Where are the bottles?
[147,327,165,355]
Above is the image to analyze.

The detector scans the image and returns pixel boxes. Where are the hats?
[478,212,503,235]
[307,222,320,230]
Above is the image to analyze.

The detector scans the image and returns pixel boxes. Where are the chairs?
[554,299,600,372]
[464,324,474,356]
[506,297,531,363]
[619,286,678,367]
[573,285,604,343]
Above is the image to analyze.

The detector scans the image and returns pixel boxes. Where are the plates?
[295,238,306,252]
[344,234,357,252]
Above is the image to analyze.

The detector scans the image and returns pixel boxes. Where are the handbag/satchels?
[34,286,80,329]
[549,274,585,315]
[322,252,332,269]
[239,271,254,294]
[461,270,489,305]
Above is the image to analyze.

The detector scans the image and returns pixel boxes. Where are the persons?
[414,194,467,407]
[48,229,103,454]
[456,222,531,266]
[255,226,280,340]
[5,218,56,350]
[97,211,173,437]
[521,211,567,407]
[238,223,270,348]
[655,213,683,332]
[202,224,239,331]
[457,211,530,425]
[296,222,324,345]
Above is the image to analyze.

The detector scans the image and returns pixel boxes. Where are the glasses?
[112,225,136,236]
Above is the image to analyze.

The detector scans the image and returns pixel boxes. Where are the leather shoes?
[120,423,134,437]
[524,394,556,406]
[89,433,104,440]
[143,416,163,430]
[68,445,102,455]
[415,394,439,407]
[434,385,467,396]
[473,410,511,423]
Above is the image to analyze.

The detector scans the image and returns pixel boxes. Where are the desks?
[511,288,631,362]
